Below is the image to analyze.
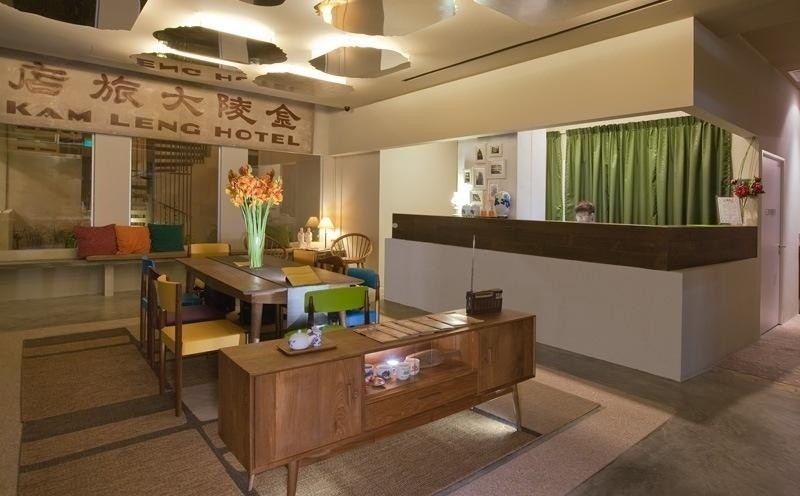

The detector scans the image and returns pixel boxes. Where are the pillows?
[72,221,119,259]
[146,222,185,252]
[112,223,152,254]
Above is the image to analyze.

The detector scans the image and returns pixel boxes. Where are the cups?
[462,204,480,217]
[365,358,420,388]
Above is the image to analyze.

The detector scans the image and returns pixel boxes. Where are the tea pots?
[287,329,316,350]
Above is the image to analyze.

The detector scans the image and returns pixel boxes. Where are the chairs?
[241,231,291,262]
[141,257,249,417]
[330,231,374,267]
[317,253,347,280]
[329,267,380,326]
[293,247,317,267]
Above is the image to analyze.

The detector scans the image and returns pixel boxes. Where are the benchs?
[80,250,188,297]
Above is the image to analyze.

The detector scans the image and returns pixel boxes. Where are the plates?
[275,336,336,355]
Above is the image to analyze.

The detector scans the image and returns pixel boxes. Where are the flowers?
[729,173,764,205]
[227,162,283,268]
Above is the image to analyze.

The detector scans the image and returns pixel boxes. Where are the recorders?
[466,287,503,315]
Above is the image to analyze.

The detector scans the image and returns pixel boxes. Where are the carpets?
[10,325,600,495]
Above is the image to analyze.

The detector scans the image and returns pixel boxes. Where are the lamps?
[304,216,320,230]
[318,217,335,248]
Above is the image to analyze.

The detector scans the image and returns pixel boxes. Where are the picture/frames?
[462,138,508,204]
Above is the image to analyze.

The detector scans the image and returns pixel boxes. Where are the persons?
[575,200,595,223]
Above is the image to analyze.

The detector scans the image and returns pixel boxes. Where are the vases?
[735,195,750,225]
[297,228,305,248]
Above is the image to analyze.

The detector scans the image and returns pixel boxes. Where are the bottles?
[481,199,495,217]
[310,326,322,347]
[298,227,304,247]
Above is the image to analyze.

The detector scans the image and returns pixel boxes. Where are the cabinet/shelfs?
[215,301,537,494]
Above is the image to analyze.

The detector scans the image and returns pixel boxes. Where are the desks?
[174,250,370,343]
[286,246,347,275]
